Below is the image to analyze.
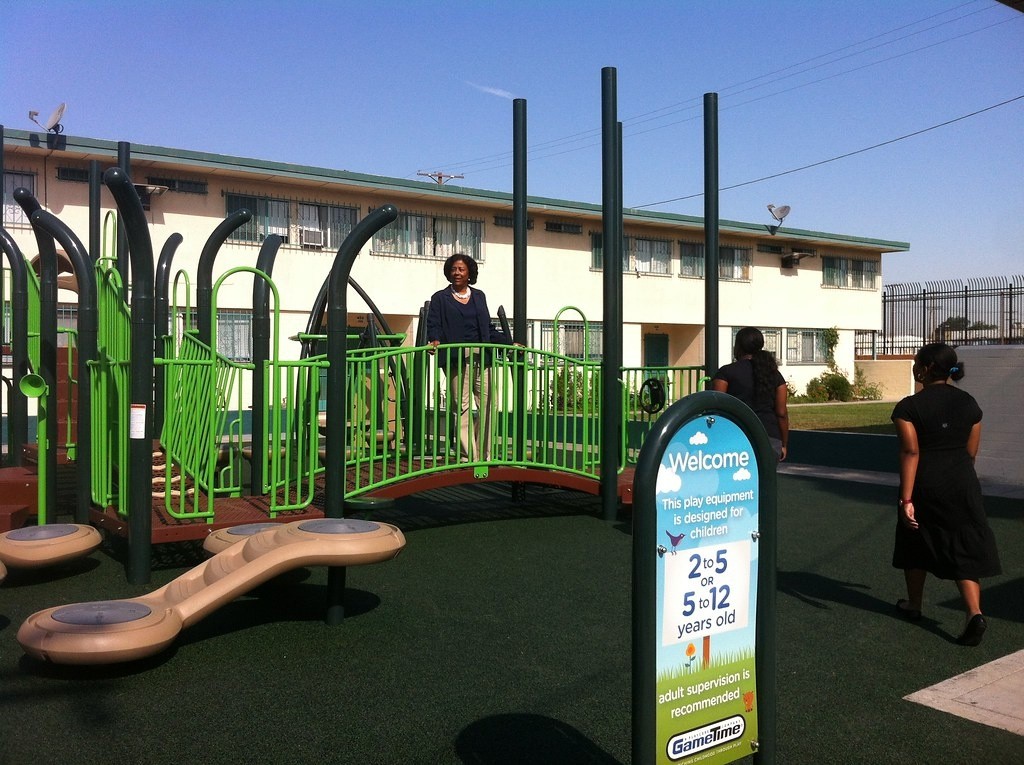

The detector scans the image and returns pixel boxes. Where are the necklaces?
[451,285,471,299]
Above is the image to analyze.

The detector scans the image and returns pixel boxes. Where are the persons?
[713,327,789,463]
[891,343,1003,646]
[426,254,526,465]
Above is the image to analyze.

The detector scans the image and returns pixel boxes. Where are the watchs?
[899,498,912,507]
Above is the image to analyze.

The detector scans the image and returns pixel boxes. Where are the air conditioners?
[299,228,324,248]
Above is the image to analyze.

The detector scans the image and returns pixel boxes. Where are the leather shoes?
[958,614,986,647]
[896,599,922,617]
